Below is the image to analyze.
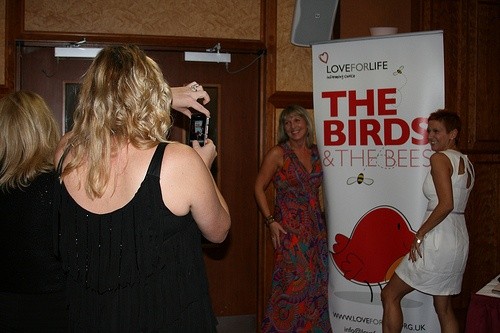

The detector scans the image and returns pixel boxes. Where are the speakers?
[291,0,338,47]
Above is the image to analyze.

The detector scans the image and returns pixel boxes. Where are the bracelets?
[415,236,422,244]
[265,215,274,227]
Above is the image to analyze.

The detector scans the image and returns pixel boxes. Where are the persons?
[254,104,332,333]
[45,44,231,333]
[381,109,475,333]
[0,89,62,333]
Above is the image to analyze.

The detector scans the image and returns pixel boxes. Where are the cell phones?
[188,112,210,148]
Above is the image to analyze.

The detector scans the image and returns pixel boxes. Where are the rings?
[412,250,415,253]
[191,84,198,91]
